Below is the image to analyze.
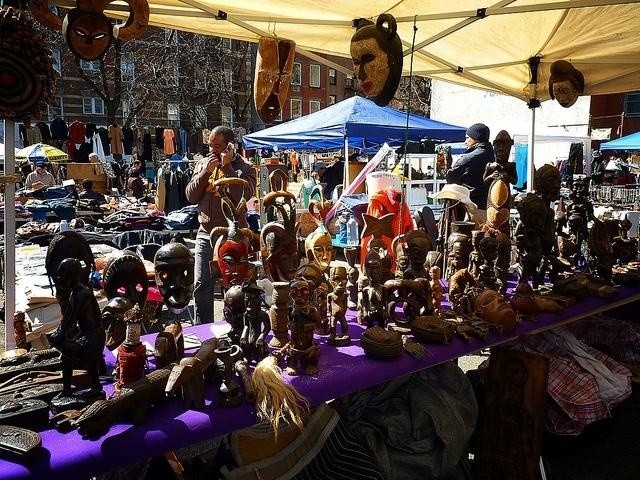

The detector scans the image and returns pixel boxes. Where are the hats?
[330,267,347,281]
[493,129,514,145]
[466,123,489,142]
[243,277,265,293]
[568,174,591,183]
[37,161,43,166]
[315,162,324,170]
[449,233,468,243]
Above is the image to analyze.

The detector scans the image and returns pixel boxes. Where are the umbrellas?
[15,142,68,160]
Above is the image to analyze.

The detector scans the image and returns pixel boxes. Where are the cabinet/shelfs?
[4,272,640,474]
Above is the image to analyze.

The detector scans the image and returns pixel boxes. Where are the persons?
[453,287,522,336]
[479,237,500,292]
[566,174,596,244]
[103,250,149,313]
[128,168,141,197]
[468,232,484,279]
[79,178,105,202]
[50,258,105,398]
[240,277,271,365]
[554,211,570,239]
[326,266,350,340]
[287,262,322,375]
[403,229,432,280]
[101,297,132,351]
[186,126,256,325]
[535,166,562,285]
[223,285,245,342]
[350,14,403,106]
[209,180,260,288]
[445,123,494,229]
[25,161,56,189]
[447,234,470,276]
[154,243,194,309]
[45,231,96,295]
[429,266,444,316]
[305,185,332,272]
[483,130,518,277]
[260,169,300,280]
[514,196,547,292]
[28,1,150,61]
[127,159,141,176]
[89,153,104,175]
[549,60,584,107]
[311,162,327,183]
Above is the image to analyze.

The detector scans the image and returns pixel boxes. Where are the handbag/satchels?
[492,326,630,436]
[571,316,638,380]
[226,403,388,480]
[339,360,478,480]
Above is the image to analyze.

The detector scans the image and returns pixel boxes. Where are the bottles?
[347,214,359,246]
[60,220,67,233]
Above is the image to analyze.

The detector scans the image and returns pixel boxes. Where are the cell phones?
[226,142,235,151]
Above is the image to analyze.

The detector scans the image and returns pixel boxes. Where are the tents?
[441,144,471,155]
[464,109,591,183]
[601,130,639,152]
[241,96,469,195]
[3,0,640,356]
